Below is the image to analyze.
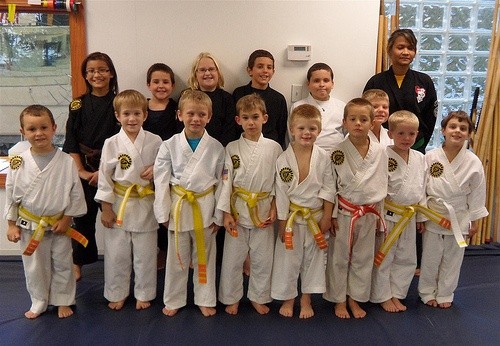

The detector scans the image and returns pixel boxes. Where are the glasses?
[196,67,217,72]
[85,68,110,76]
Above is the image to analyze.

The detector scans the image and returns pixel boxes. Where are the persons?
[225,50,289,277]
[153,90,226,316]
[141,63,185,270]
[62,52,122,280]
[370,110,429,312]
[362,29,438,275]
[5,104,88,319]
[271,103,336,319]
[324,98,389,318]
[218,94,283,314]
[179,52,233,269]
[418,110,489,307]
[285,63,346,153]
[93,90,162,309]
[362,89,395,150]
